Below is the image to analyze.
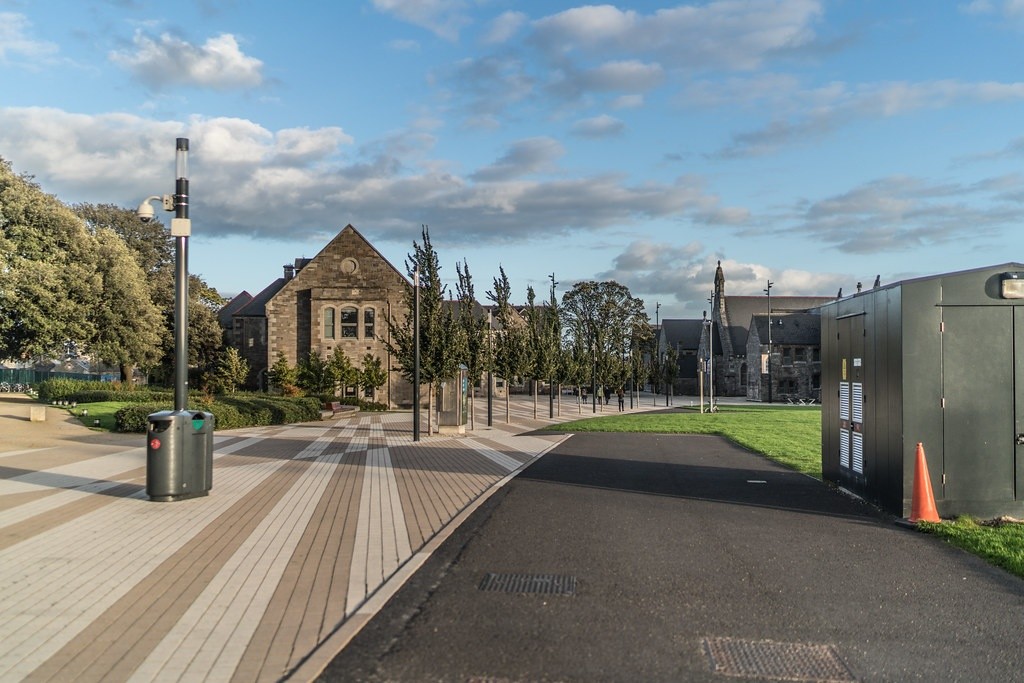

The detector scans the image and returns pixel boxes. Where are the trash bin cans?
[146,410,215,502]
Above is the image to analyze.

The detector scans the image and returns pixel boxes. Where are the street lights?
[487,309,493,427]
[665,353,669,406]
[591,341,596,414]
[547,272,559,303]
[655,302,662,329]
[762,279,774,403]
[630,348,634,409]
[702,321,715,413]
[707,290,717,325]
[413,265,421,442]
[171,136,188,411]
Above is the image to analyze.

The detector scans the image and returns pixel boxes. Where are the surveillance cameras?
[137,196,165,222]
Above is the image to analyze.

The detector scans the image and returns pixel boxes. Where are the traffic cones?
[907,441,944,524]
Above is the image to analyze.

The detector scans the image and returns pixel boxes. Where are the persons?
[582,387,588,404]
[597,385,604,404]
[618,389,625,411]
[575,386,581,404]
[604,387,610,404]
